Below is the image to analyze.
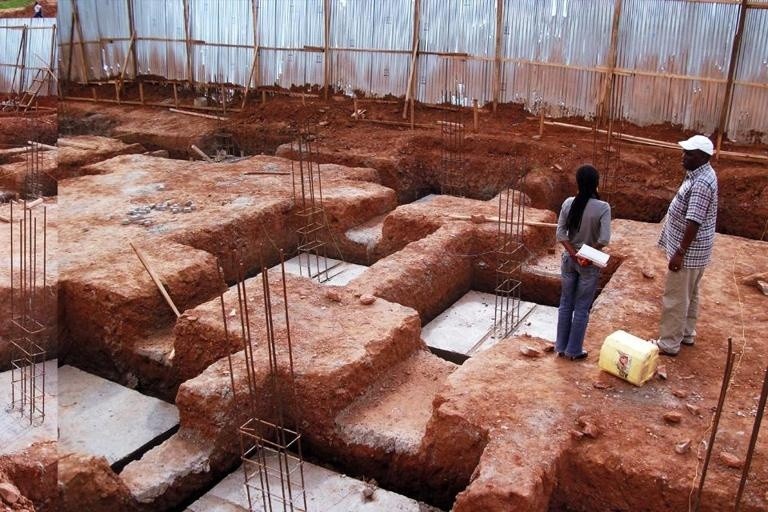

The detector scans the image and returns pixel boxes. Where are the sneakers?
[557,350,588,359]
[681,335,696,344]
[646,339,680,356]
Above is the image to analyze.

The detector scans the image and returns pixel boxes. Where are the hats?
[677,135,714,157]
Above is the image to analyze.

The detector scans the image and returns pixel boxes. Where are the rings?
[674,267,677,270]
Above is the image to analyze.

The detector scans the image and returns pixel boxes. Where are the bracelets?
[676,247,685,257]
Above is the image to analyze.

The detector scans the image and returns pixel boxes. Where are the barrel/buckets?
[598,330,659,386]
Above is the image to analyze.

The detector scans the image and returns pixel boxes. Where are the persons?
[33,1,43,17]
[649,135,719,355]
[553,164,611,360]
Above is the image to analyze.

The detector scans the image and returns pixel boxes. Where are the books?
[575,243,610,269]
